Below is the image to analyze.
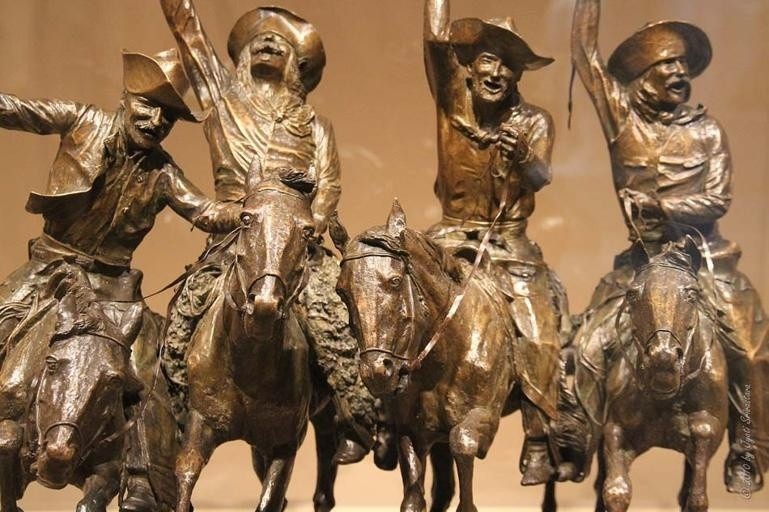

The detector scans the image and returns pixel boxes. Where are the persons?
[161,1,376,464]
[570,1,768,498]
[0,46,247,512]
[420,0,565,488]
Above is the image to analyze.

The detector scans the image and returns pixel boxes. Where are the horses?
[327,196,572,512]
[173,154,341,511]
[573,231,729,512]
[0,269,167,511]
